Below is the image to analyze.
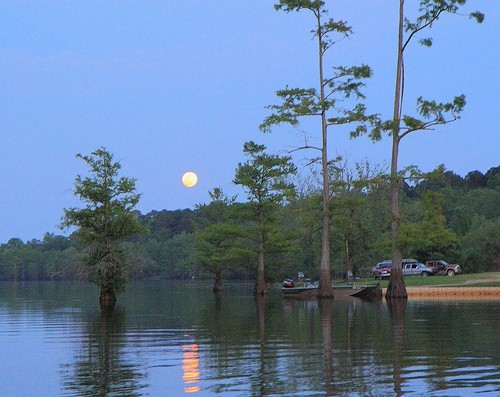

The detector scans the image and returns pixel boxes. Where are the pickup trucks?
[402,263,437,277]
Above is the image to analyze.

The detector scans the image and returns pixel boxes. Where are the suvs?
[426,261,461,277]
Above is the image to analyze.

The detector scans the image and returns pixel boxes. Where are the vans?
[297,272,304,280]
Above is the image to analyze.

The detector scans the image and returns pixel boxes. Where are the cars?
[347,271,353,277]
[282,279,294,288]
[373,263,392,279]
